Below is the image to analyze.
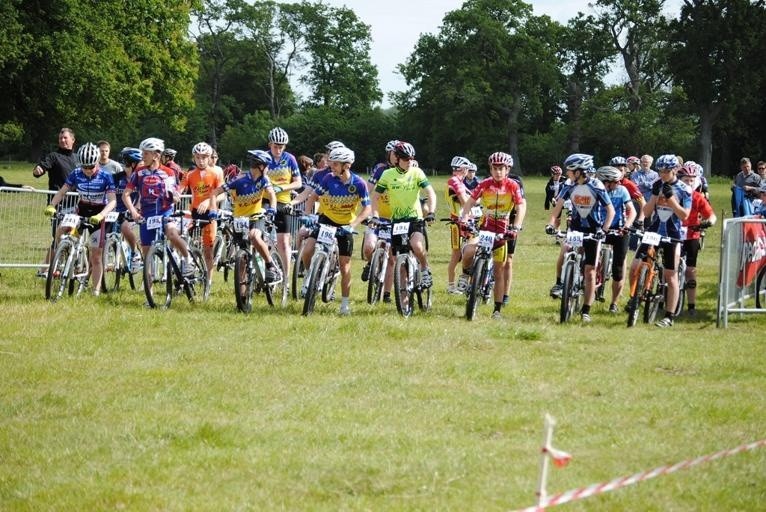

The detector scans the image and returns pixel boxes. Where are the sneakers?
[551,285,696,328]
[447,275,508,319]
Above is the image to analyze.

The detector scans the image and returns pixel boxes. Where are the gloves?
[207,210,218,218]
[341,225,353,236]
[545,224,556,234]
[506,230,517,239]
[594,229,607,240]
[44,205,56,217]
[266,208,278,216]
[653,180,673,198]
[89,213,104,225]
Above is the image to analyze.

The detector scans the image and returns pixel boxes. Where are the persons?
[544,156,717,329]
[729,157,765,217]
[32,125,526,317]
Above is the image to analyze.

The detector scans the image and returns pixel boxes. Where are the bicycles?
[439,217,522,321]
[549,210,697,328]
[44,209,287,314]
[290,208,359,317]
[360,215,433,319]
